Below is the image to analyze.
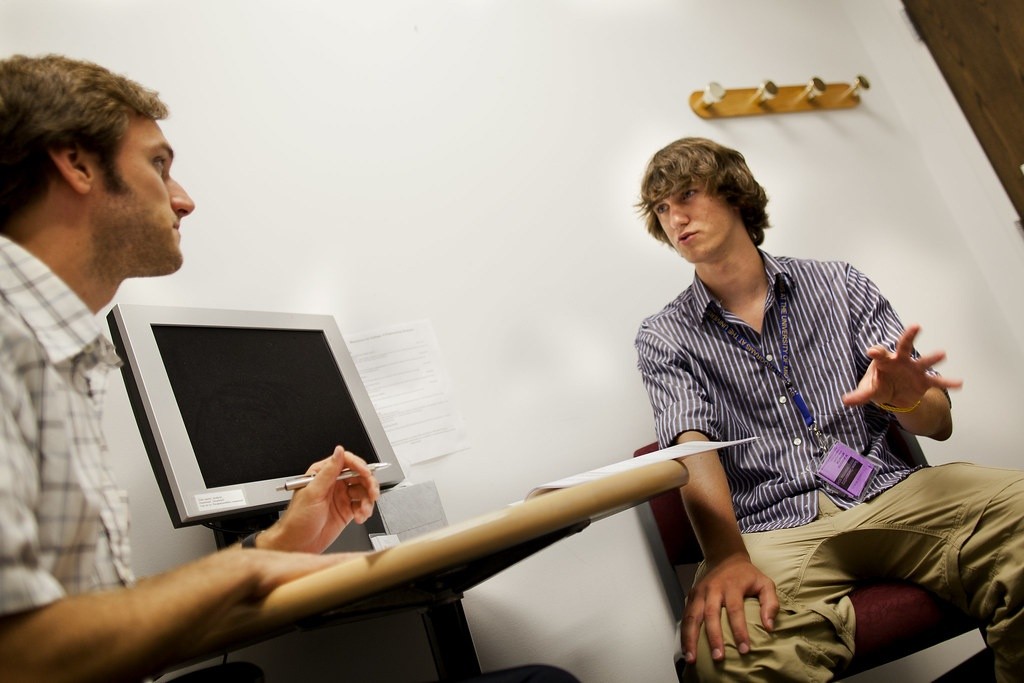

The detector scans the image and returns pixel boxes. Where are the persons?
[632,134,1024,683]
[0,46,593,683]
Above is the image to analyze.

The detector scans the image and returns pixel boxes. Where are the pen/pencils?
[274,462,394,492]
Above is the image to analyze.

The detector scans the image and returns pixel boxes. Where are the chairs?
[634,426,998,683]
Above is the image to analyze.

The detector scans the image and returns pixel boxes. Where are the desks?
[145,457,691,681]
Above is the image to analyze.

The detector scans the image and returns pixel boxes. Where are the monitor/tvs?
[105,303,405,529]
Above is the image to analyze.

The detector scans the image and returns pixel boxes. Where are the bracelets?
[866,387,922,416]
[240,526,269,556]
